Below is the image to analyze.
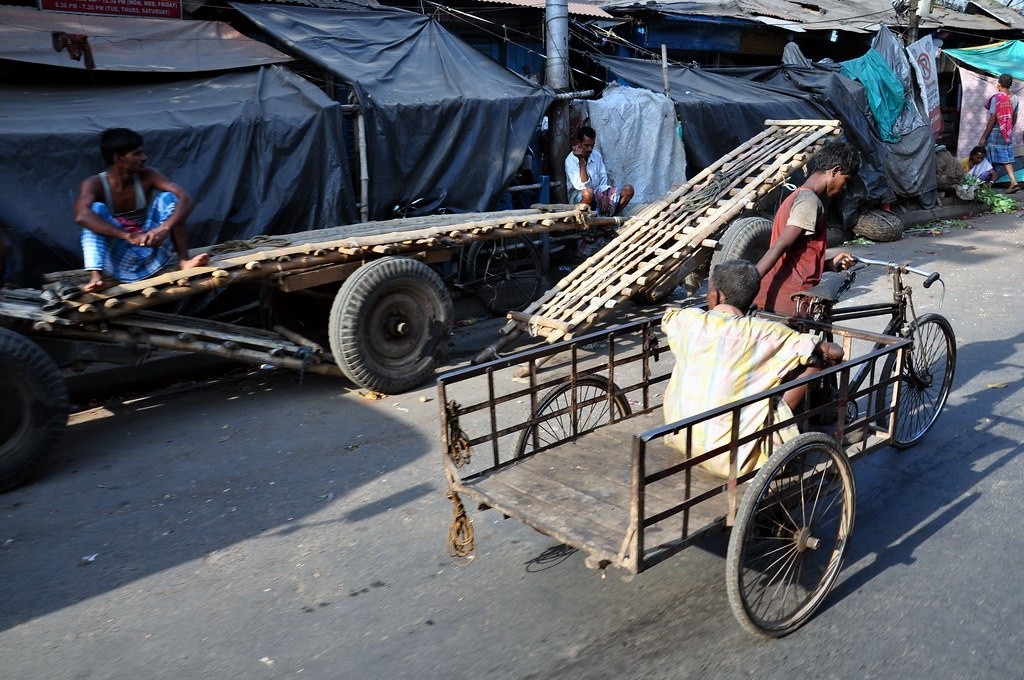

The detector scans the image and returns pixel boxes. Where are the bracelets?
[988,180,994,185]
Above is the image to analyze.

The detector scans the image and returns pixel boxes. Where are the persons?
[750,139,861,329]
[564,125,634,243]
[73,126,211,292]
[958,145,998,189]
[660,259,845,480]
[976,72,1021,194]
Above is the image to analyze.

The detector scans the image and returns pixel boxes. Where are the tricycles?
[434,251,959,643]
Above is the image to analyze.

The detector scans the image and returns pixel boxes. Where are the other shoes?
[597,226,619,240]
[582,234,594,244]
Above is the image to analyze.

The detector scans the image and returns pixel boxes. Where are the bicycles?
[382,188,542,318]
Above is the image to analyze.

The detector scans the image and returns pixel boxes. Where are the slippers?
[1005,186,1021,193]
